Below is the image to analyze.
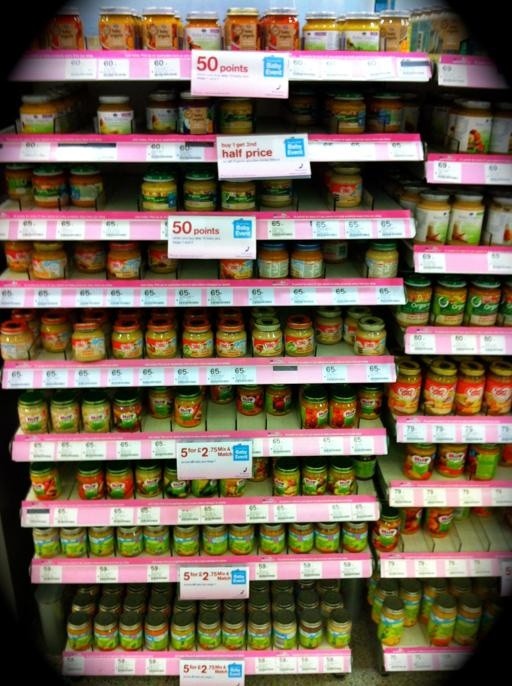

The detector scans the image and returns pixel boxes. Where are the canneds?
[44,5,470,57]
[20,85,512,155]
[66,577,353,652]
[33,507,501,558]
[365,576,500,647]
[0,163,512,434]
[30,442,377,502]
[402,443,512,481]
